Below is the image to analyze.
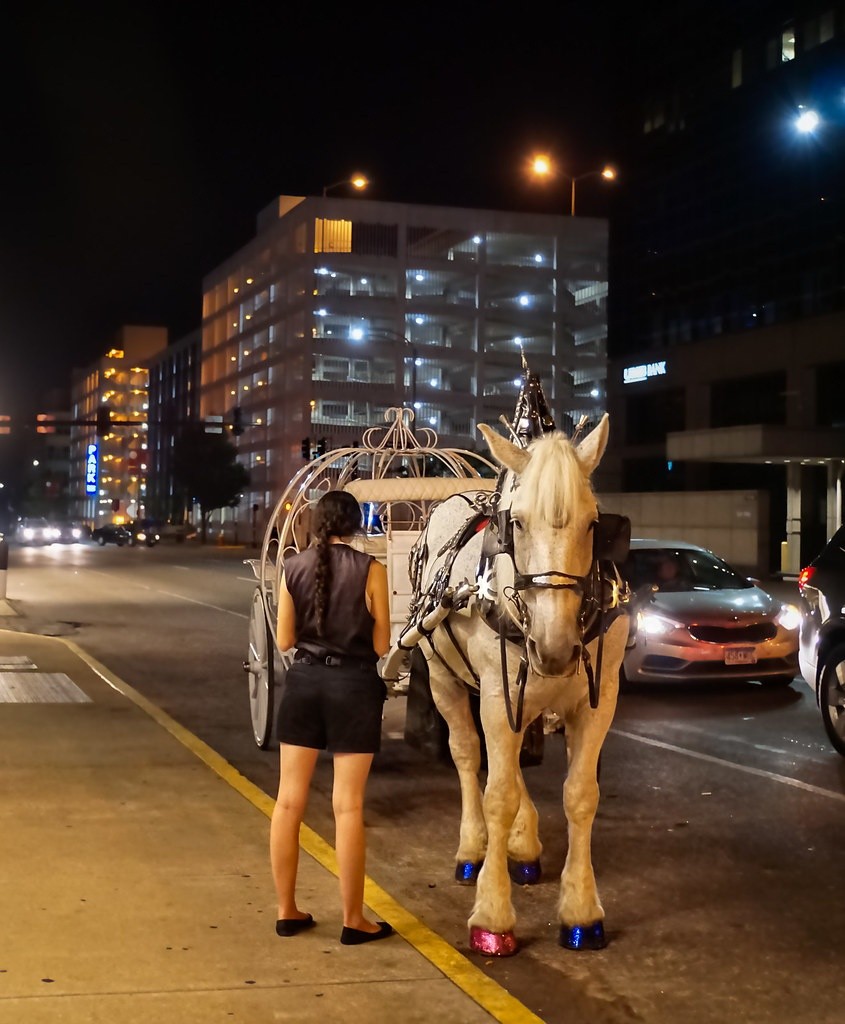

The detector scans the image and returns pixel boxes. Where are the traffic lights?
[302,437,311,460]
[316,437,325,462]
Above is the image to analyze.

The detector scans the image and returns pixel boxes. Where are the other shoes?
[340,922,392,944]
[275,912,312,936]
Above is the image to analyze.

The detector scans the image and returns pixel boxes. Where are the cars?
[624,536,801,692]
[14,516,160,549]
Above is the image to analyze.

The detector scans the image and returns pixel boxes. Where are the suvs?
[798,523,845,752]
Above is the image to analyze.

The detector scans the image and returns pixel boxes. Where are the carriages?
[239,348,630,962]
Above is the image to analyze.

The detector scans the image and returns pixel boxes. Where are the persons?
[269,490,393,948]
[652,551,679,592]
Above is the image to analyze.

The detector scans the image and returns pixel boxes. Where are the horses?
[414,412,636,958]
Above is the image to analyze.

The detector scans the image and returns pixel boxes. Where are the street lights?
[528,154,622,216]
[351,324,419,449]
[319,172,371,197]
